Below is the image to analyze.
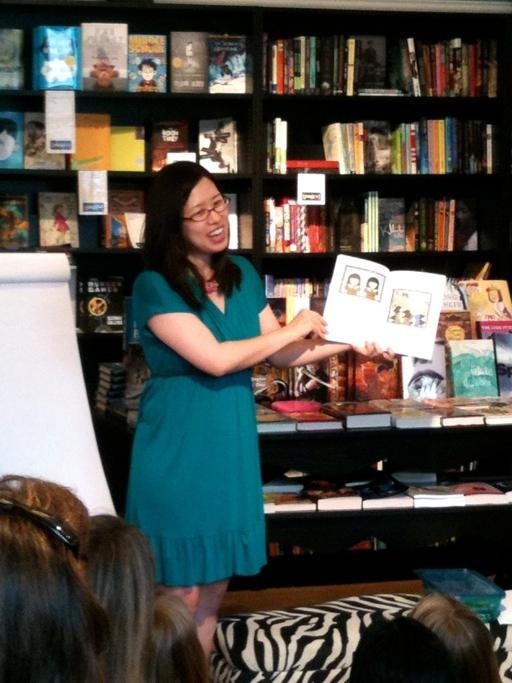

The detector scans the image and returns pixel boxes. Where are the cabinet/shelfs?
[2,0,512,577]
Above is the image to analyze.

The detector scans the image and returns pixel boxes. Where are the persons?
[410,591,502,683]
[123,161,397,666]
[345,617,456,683]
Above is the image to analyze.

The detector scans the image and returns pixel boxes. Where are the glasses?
[0,496,80,566]
[183,197,230,222]
